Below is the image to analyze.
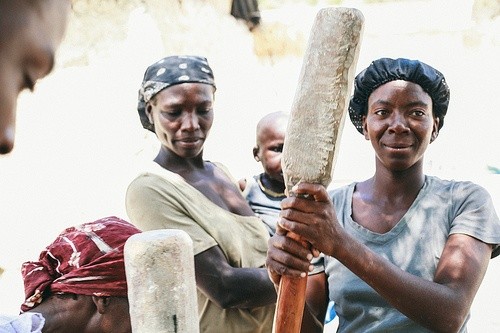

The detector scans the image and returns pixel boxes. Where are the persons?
[0,0,71,155]
[239,111,291,239]
[265,58,500,333]
[0,217,143,333]
[125,56,277,332]
